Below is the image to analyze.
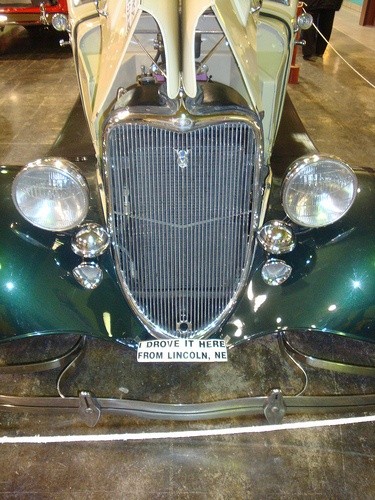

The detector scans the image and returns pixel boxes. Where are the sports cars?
[2,0,375,442]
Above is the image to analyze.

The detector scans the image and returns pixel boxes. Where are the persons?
[298,0,343,62]
